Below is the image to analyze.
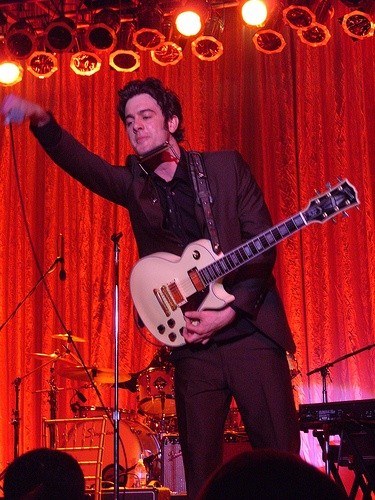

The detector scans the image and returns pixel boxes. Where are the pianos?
[297,398,375,500]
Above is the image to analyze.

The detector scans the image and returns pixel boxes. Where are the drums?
[225,353,301,439]
[61,417,162,491]
[144,415,180,438]
[137,362,179,416]
[75,404,135,422]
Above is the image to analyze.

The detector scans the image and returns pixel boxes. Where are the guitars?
[130,176,362,346]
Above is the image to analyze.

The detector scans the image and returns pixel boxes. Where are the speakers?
[101,488,158,500]
[161,436,187,493]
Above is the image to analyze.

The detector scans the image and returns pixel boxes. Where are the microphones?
[74,389,88,403]
[60,236,66,281]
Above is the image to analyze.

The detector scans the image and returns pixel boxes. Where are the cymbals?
[25,332,131,383]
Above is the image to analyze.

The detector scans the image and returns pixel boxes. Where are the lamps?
[0,0,375,87]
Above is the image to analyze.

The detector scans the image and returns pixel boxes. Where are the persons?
[1,76,302,500]
[195,449,347,500]
[0,447,86,500]
